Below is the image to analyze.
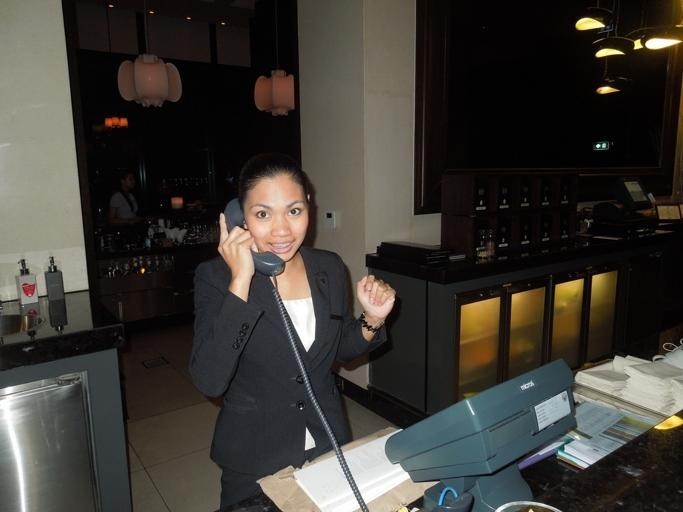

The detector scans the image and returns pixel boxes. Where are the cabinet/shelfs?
[370,248,628,430]
[445,166,581,260]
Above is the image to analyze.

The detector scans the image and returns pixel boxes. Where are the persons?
[108,170,145,225]
[187,152,397,512]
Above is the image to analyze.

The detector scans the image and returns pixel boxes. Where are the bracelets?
[359,312,381,333]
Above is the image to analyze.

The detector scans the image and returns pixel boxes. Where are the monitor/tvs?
[385,358,577,512]
[618,177,652,220]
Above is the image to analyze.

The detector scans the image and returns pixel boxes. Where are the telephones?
[223,198,285,276]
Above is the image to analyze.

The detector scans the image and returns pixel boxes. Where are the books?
[558,417,652,469]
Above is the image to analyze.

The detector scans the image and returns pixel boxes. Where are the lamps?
[252,0,298,117]
[574,0,683,96]
[117,1,183,109]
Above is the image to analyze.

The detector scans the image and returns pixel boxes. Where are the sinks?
[0,314,45,337]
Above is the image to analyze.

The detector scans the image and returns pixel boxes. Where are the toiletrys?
[44,255,66,301]
[20,305,41,344]
[14,259,39,305]
[49,300,68,335]
